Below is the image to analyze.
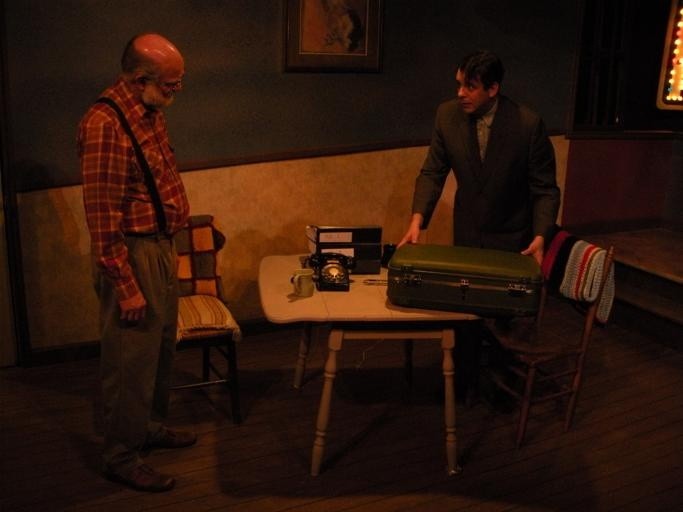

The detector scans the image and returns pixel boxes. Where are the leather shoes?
[100,464,175,493]
[155,425,196,447]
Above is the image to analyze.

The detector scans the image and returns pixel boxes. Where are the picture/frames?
[275,1,386,76]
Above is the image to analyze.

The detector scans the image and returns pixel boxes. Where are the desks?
[254,248,480,481]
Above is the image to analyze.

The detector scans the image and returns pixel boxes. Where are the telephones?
[308,252,357,292]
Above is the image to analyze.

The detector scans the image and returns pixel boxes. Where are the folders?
[304,223,383,275]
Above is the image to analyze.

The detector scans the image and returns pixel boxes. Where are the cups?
[291,268,315,298]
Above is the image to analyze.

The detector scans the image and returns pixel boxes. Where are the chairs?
[482,218,619,456]
[160,206,246,429]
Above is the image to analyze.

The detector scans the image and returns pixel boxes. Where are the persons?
[397,47,561,404]
[77,33,197,493]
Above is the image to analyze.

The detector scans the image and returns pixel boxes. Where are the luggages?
[387,241,544,319]
